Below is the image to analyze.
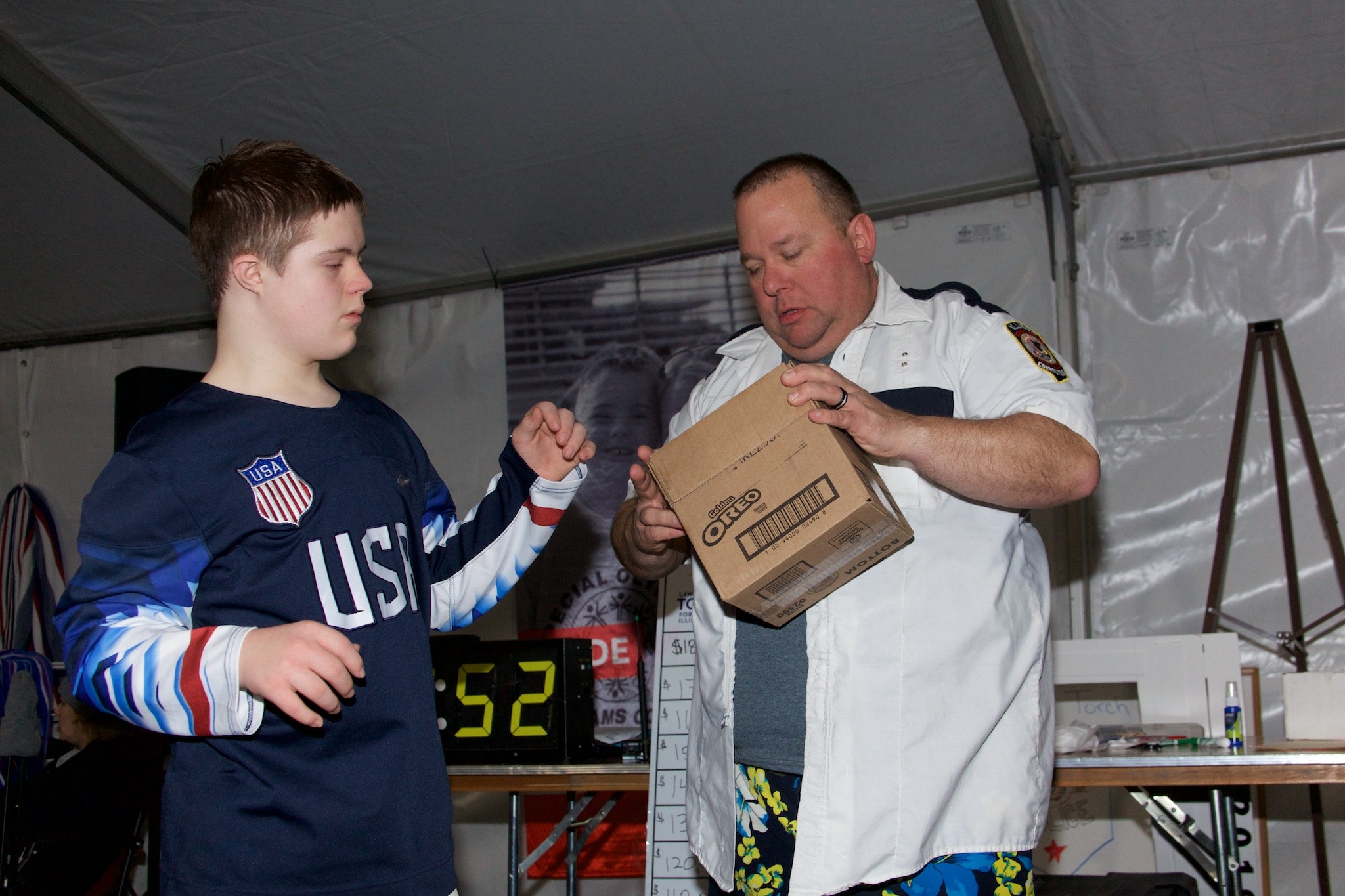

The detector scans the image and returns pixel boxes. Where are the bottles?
[1224,682,1242,748]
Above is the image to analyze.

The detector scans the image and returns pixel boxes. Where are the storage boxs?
[647,360,914,628]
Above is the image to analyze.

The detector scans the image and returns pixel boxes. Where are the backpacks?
[0,649,55,756]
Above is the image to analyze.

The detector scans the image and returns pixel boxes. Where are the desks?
[448,740,1345,896]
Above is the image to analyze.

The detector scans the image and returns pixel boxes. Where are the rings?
[831,387,848,409]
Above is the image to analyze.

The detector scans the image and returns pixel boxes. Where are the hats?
[58,677,95,714]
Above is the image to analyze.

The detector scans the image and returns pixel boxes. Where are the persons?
[7,676,148,896]
[56,141,596,896]
[522,342,667,628]
[610,153,1101,896]
[658,342,725,438]
